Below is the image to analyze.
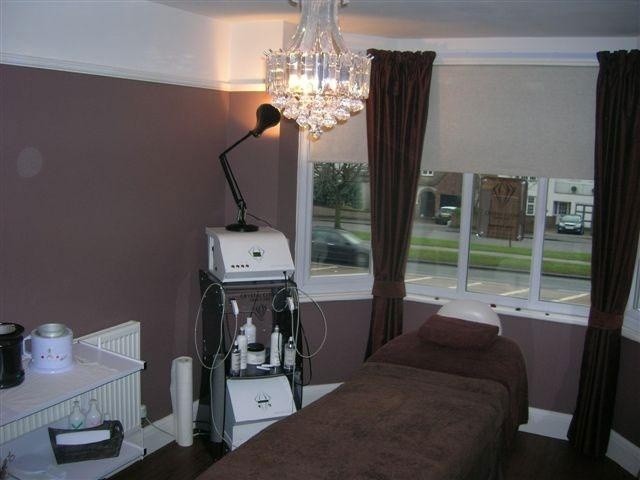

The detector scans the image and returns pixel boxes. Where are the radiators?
[0,320,141,445]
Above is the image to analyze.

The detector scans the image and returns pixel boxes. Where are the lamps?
[265,1,374,139]
[218,105,281,231]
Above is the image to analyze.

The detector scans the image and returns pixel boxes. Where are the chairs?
[437,298,502,335]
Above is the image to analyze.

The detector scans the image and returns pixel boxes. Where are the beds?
[191,324,524,478]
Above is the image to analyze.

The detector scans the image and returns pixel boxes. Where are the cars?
[437,206,457,224]
[558,215,584,234]
[312,221,369,268]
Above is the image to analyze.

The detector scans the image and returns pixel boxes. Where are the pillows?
[419,315,500,350]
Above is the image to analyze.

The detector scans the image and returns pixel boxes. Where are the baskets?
[47,419,124,464]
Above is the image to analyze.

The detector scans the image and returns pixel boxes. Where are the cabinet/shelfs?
[1,339,147,480]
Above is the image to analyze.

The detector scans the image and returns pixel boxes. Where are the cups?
[0,323,73,389]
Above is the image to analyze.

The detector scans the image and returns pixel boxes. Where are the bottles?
[231,317,295,374]
